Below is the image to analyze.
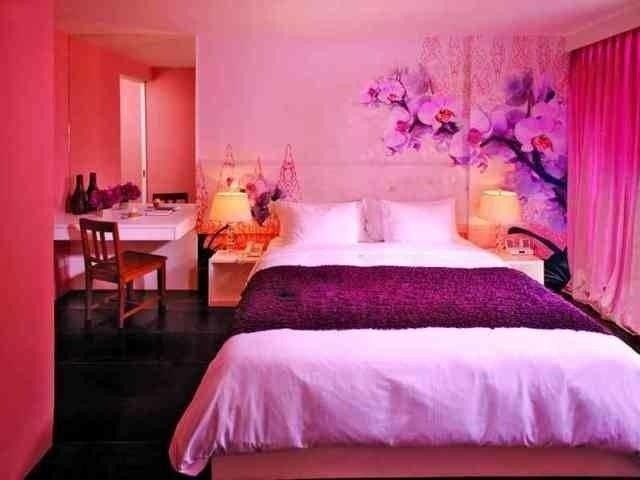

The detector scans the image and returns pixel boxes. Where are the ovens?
[209,165,640,478]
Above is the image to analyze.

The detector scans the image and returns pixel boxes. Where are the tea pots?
[65,31,199,211]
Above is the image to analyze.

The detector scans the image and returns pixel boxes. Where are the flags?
[119,202,129,208]
[96,208,113,217]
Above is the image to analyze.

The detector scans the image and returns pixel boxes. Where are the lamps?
[78,219,168,329]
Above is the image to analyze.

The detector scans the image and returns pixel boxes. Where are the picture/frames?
[54,211,199,291]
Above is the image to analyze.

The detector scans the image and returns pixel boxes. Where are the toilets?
[245,241,264,257]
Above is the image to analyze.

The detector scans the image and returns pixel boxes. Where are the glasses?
[478,188,521,255]
[208,192,252,259]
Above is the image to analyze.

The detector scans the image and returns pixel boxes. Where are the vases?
[90,189,116,209]
[116,182,141,202]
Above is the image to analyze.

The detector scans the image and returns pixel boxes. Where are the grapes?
[487,247,545,285]
[207,250,267,309]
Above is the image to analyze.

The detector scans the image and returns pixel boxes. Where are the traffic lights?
[268,197,456,244]
